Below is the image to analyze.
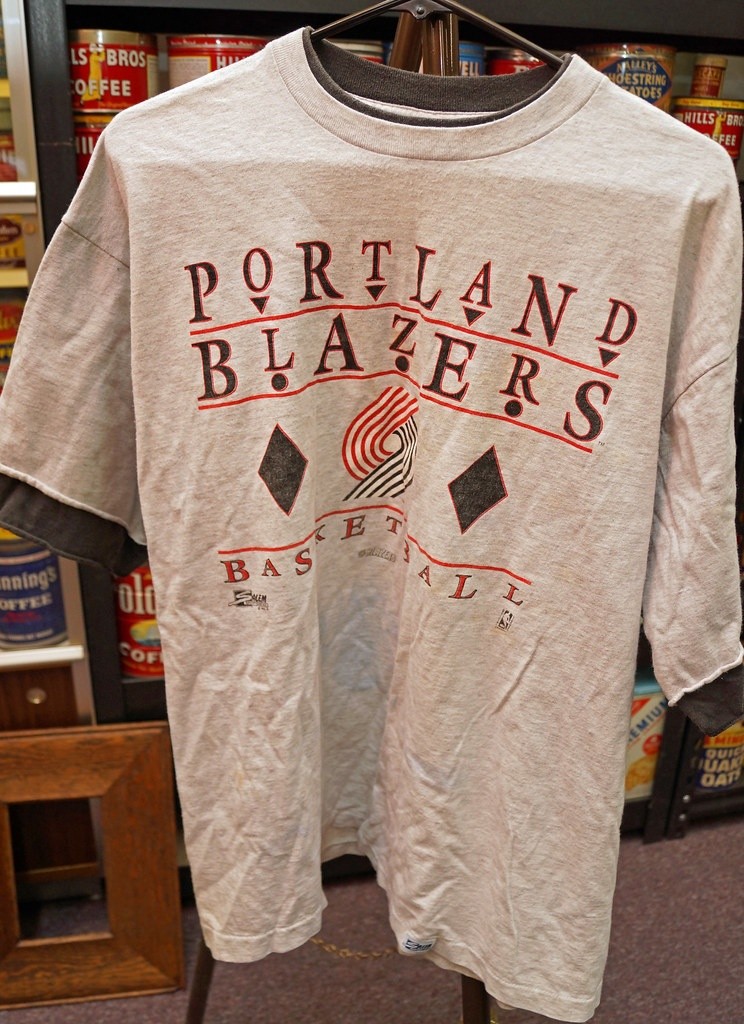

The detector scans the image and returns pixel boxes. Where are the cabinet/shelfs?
[0,0,744,908]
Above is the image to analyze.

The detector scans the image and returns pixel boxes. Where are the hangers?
[311,0,563,71]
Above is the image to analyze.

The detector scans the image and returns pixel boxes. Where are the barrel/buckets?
[623,680,667,798]
[71,26,744,198]
[0,526,69,649]
[697,715,744,788]
[118,556,163,682]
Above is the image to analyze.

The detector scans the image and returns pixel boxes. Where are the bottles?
[0,298,25,393]
[691,54,727,98]
[0,215,26,269]
[0,76,16,181]
